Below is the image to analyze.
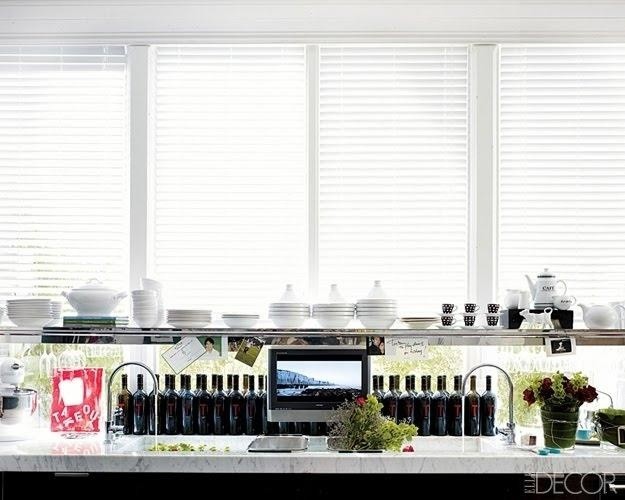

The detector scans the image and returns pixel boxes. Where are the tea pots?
[574,301,625,330]
[524,268,570,309]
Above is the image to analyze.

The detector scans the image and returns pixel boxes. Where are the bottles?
[116,374,500,438]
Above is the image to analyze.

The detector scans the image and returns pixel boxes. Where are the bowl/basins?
[141,278,168,326]
[133,289,158,328]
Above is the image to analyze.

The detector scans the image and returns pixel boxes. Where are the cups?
[577,429,592,440]
[441,301,503,331]
[501,286,531,310]
[551,295,577,309]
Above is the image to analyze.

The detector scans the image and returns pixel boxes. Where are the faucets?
[460,363,517,444]
[596,390,614,409]
[105,361,159,443]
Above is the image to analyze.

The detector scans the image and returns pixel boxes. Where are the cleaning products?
[50,366,104,432]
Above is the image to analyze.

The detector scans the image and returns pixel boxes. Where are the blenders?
[0,358,40,442]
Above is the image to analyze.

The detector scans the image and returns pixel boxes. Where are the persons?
[202,337,218,355]
[368,337,382,354]
[379,341,384,354]
[243,340,253,354]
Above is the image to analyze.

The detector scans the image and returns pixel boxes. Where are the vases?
[537,401,581,454]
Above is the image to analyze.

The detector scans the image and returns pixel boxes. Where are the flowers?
[320,393,420,452]
[517,364,599,415]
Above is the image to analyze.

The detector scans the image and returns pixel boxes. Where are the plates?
[354,299,399,328]
[269,302,311,327]
[400,317,437,329]
[165,308,212,328]
[221,313,260,326]
[3,291,62,332]
[310,304,355,327]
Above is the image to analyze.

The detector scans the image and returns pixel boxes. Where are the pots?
[64,278,131,314]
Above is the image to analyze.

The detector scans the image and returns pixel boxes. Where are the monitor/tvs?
[266,344,371,423]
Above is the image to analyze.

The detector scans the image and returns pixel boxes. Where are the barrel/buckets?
[540,407,579,450]
[1,388,36,441]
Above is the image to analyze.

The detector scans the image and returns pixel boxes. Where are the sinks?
[122,435,258,452]
[307,436,329,453]
[401,435,501,453]
